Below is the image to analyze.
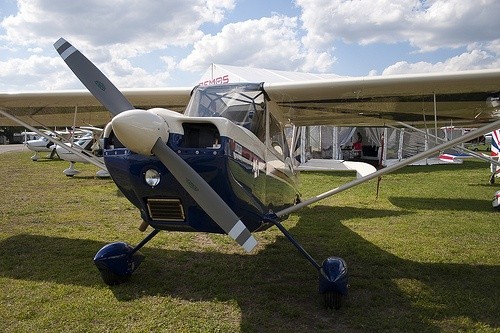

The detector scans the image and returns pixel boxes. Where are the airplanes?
[461,131,493,152]
[0,37,500,310]
[20,129,84,161]
[49,126,111,178]
